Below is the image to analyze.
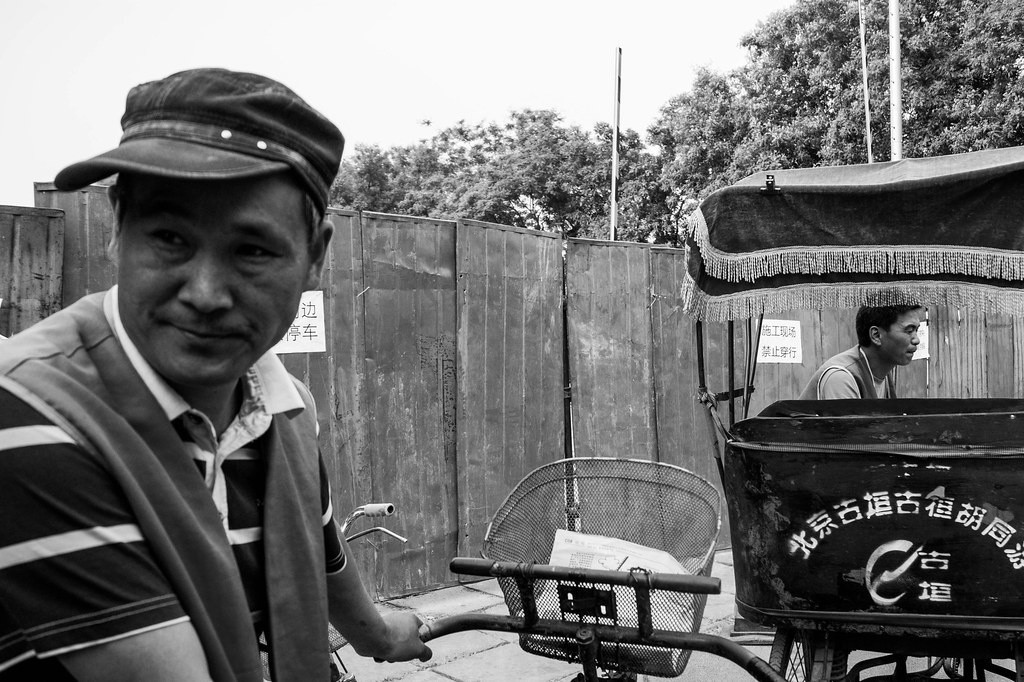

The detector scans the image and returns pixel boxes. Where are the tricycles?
[378,457,798,682]
[253,502,411,682]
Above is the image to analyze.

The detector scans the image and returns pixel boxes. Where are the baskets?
[480,454,724,680]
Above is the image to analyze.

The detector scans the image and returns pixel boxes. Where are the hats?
[51,68,344,214]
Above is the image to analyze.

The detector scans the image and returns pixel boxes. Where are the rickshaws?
[678,145,1024,680]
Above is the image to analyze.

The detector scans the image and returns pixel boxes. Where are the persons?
[2,64,435,682]
[801,287,929,399]
